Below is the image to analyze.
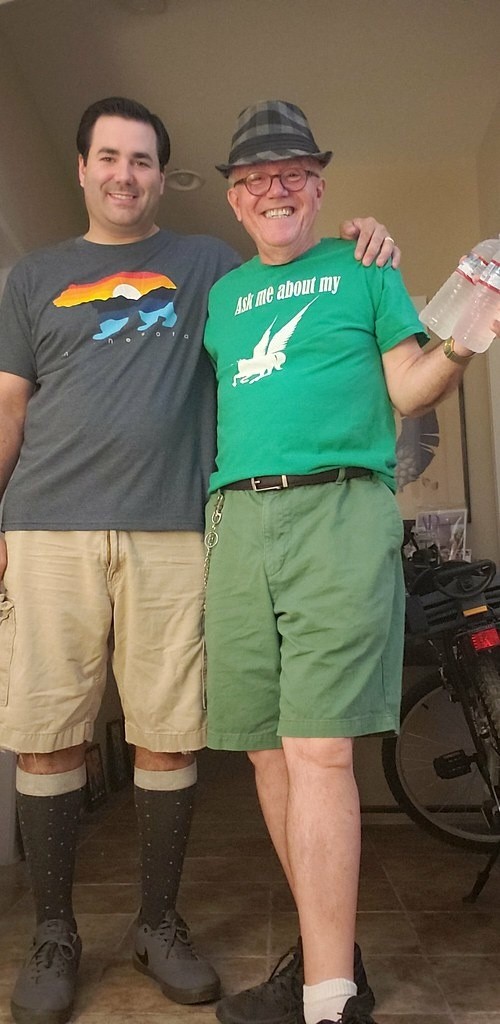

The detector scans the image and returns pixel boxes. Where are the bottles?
[452,250,500,354]
[418,239,499,341]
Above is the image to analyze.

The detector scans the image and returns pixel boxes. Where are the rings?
[385,237,394,243]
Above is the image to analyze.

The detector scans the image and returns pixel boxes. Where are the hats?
[214,100,333,179]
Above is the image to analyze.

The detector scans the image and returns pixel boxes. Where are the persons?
[203,100,500,1024]
[0,96,402,1024]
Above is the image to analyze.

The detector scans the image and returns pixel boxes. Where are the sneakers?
[131,908,221,1004]
[10,930,82,1024]
[316,995,377,1024]
[216,934,375,1024]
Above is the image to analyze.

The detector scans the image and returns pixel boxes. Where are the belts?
[220,466,372,492]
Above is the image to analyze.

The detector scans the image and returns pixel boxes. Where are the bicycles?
[379,528,499,902]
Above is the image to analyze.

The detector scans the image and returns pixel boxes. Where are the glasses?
[233,167,320,196]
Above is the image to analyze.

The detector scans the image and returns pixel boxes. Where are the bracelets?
[444,337,476,366]
